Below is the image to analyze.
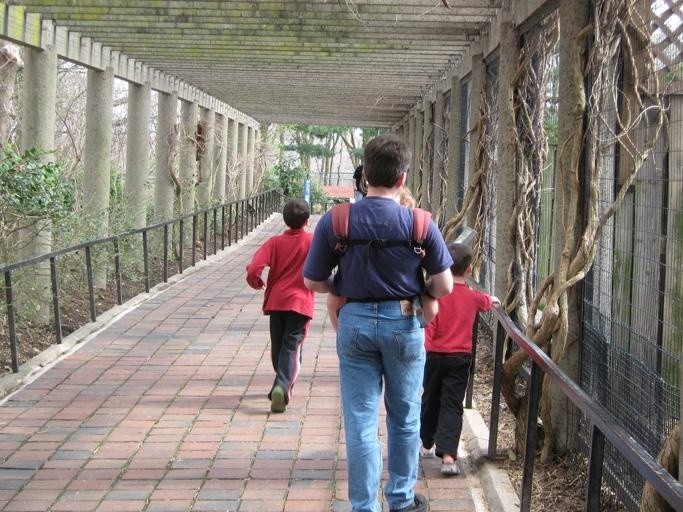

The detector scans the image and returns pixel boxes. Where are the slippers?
[439,459,461,476]
[418,441,436,459]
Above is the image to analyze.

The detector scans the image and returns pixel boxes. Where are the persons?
[420,243,501,476]
[326,185,440,333]
[301,132,454,511]
[246,199,317,413]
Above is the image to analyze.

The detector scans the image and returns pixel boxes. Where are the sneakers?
[387,492,428,512]
[269,383,288,414]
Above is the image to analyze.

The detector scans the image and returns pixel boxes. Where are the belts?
[345,294,416,305]
